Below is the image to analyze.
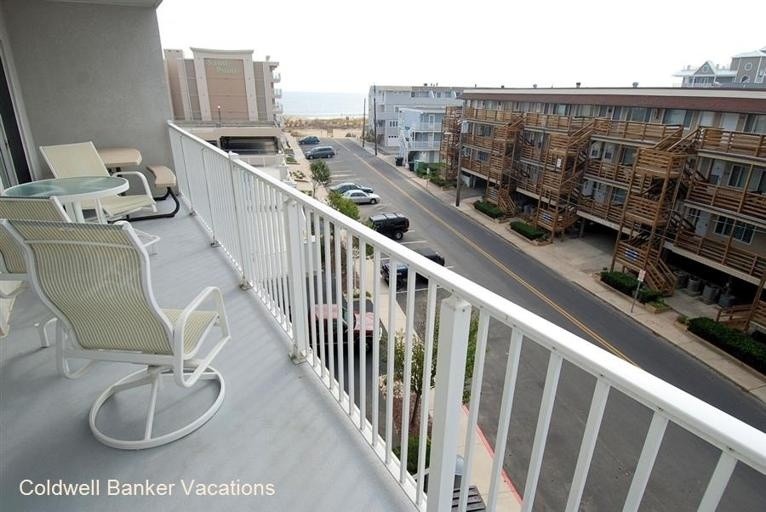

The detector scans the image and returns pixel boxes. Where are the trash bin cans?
[395,157,403,166]
[409,163,414,171]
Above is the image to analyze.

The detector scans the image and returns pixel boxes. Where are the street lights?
[217,105,221,121]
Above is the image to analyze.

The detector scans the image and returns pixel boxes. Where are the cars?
[330,182,373,194]
[304,145,336,160]
[299,135,320,144]
[289,303,382,355]
[340,189,381,205]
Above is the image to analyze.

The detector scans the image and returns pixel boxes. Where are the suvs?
[369,212,409,240]
[381,248,445,292]
